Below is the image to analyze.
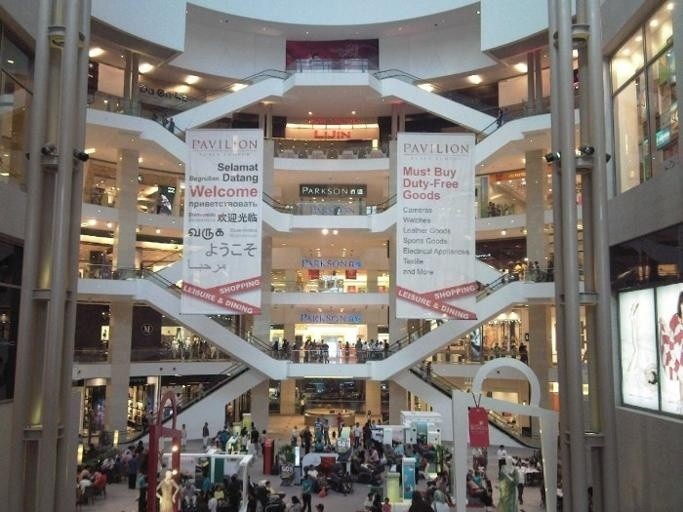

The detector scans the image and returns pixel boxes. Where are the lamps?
[74,147,89,161]
[41,142,57,155]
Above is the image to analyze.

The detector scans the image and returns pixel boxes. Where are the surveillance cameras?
[73,149,89,161]
[41,143,55,154]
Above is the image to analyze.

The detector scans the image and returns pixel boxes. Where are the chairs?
[75,486,108,512]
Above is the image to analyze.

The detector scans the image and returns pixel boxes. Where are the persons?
[155,193,162,215]
[272,338,389,364]
[493,342,528,363]
[151,114,156,121]
[177,340,208,359]
[476,260,554,295]
[74,412,562,512]
[161,113,167,128]
[487,201,514,218]
[660,293,682,404]
[167,118,174,134]
